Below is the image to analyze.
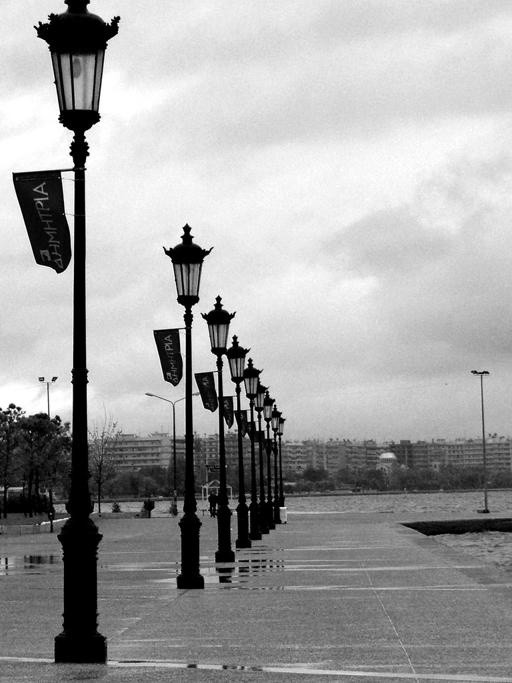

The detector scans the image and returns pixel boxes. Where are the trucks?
[0,484,56,501]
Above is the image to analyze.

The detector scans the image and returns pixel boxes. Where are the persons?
[208,490,217,518]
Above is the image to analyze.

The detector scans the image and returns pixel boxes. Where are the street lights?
[226,330,257,549]
[144,389,203,514]
[160,222,214,592]
[31,0,130,670]
[242,354,289,539]
[196,292,238,563]
[36,374,59,419]
[470,369,492,513]
